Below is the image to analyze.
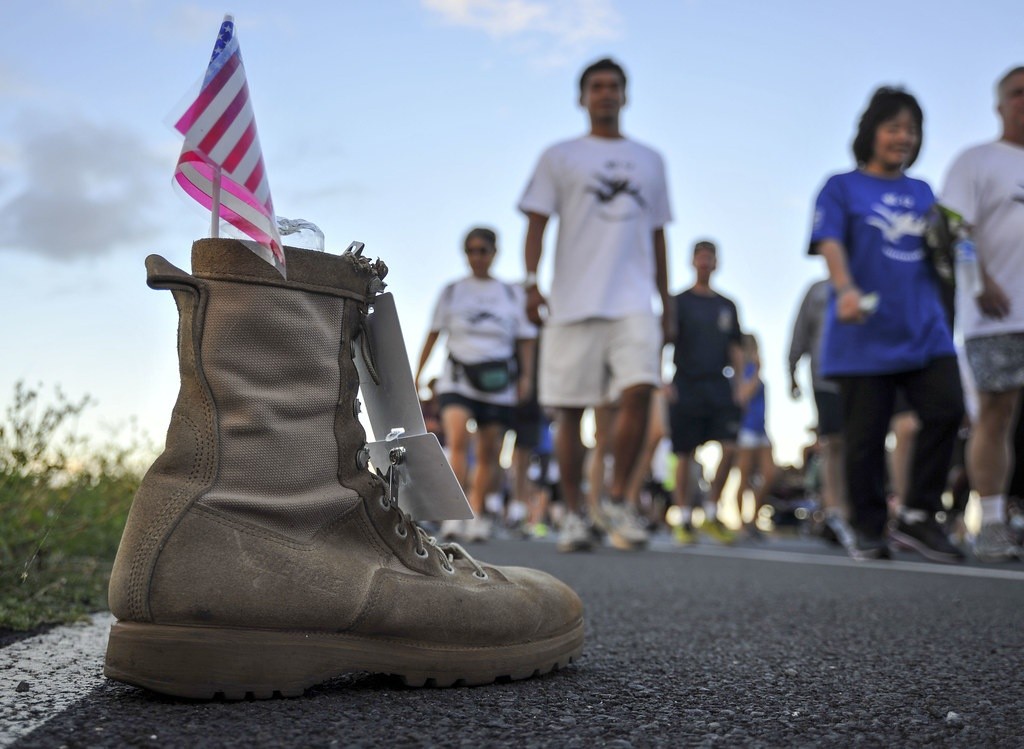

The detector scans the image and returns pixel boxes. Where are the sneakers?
[420,477,1024,566]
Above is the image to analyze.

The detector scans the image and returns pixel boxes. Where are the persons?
[415,227,773,542]
[787,65,1024,565]
[518,61,680,553]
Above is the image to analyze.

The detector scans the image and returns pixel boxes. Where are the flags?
[172,14,288,277]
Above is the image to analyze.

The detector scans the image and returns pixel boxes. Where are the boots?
[103,235,587,695]
